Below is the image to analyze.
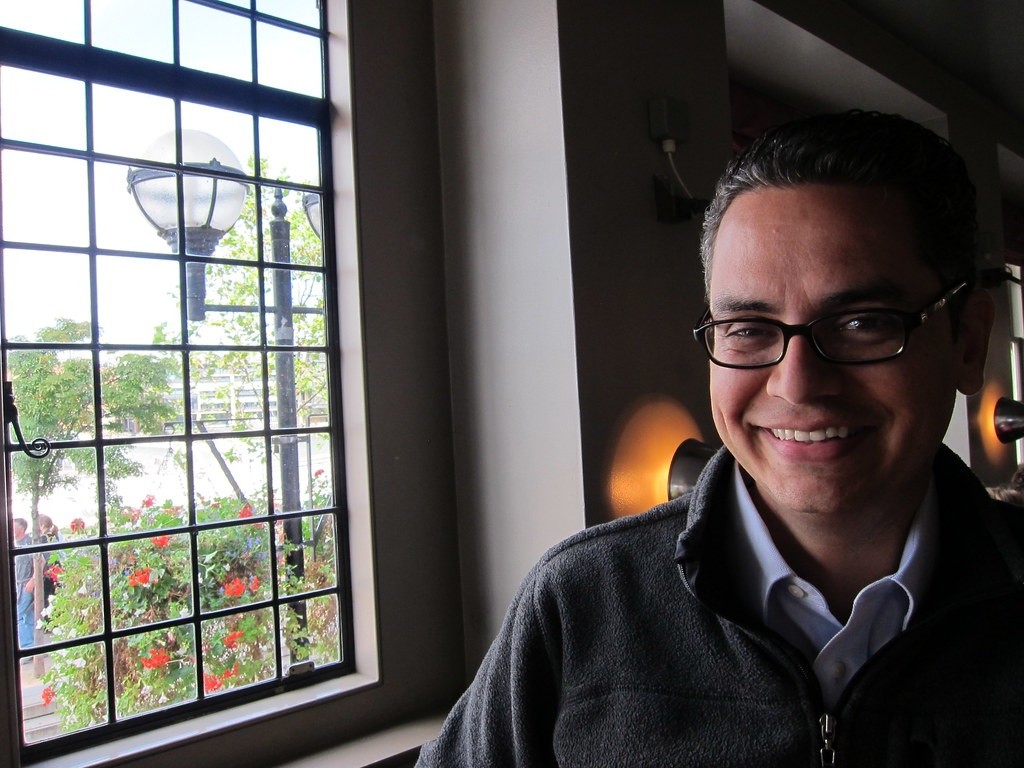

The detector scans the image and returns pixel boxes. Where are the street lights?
[124,157,324,666]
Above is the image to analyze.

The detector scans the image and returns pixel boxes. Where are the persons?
[414,108,1024,768]
[36,516,61,633]
[12,518,46,664]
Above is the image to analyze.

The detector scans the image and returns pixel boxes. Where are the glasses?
[694,277,969,371]
